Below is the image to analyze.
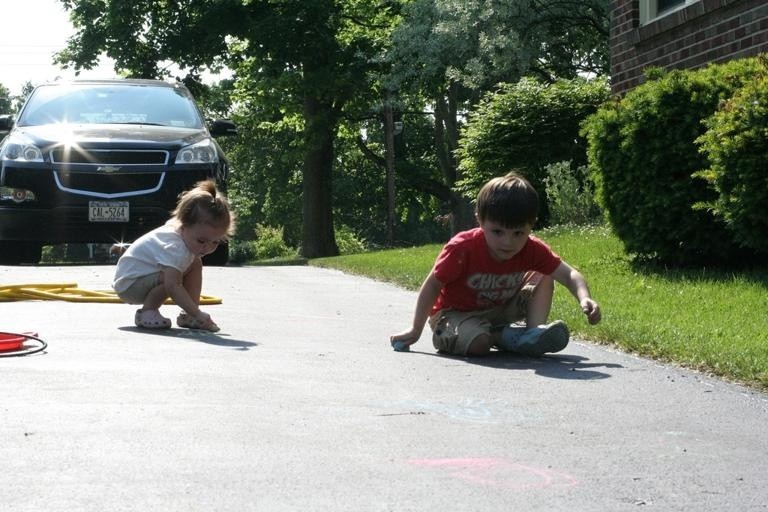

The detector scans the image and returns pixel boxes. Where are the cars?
[0,78,238,266]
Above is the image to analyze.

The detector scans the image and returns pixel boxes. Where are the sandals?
[135,309,171,328]
[177,312,209,328]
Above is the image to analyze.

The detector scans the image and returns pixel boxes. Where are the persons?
[110,180,236,332]
[388,171,603,360]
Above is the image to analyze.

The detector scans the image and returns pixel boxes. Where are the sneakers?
[498,320,570,358]
